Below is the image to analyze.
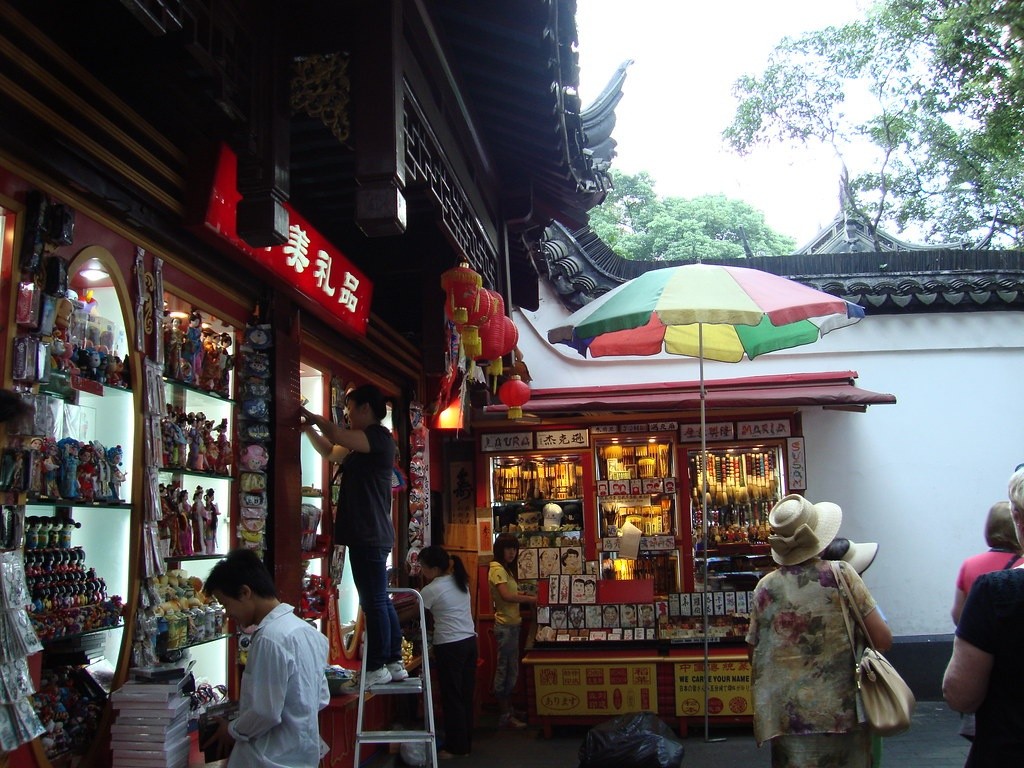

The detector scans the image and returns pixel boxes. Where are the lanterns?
[441,257,531,418]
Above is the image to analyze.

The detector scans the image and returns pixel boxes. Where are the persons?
[394,545,476,759]
[157,300,234,556]
[744,494,891,768]
[23,436,128,641]
[33,670,103,759]
[302,385,407,692]
[819,536,883,768]
[202,545,331,768]
[950,501,1024,741]
[941,462,1024,768]
[486,534,539,731]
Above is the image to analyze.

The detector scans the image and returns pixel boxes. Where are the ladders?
[353,588,438,768]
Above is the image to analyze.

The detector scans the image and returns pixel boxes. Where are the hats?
[768,494,843,566]
[840,538,878,574]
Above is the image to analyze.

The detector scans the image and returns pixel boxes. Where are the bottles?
[155,602,222,650]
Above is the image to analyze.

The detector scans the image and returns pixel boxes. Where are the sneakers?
[386,660,408,680]
[496,715,527,730]
[510,706,527,718]
[340,663,392,693]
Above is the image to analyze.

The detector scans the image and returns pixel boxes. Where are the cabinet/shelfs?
[25,363,131,639]
[163,377,233,649]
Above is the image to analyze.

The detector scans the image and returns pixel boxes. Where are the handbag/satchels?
[831,561,915,737]
[958,714,975,742]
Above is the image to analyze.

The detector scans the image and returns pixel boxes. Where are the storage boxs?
[109,663,194,768]
[14,186,74,383]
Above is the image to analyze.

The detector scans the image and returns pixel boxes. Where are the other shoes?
[438,750,468,759]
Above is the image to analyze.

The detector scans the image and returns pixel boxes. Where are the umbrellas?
[547,255,864,744]
